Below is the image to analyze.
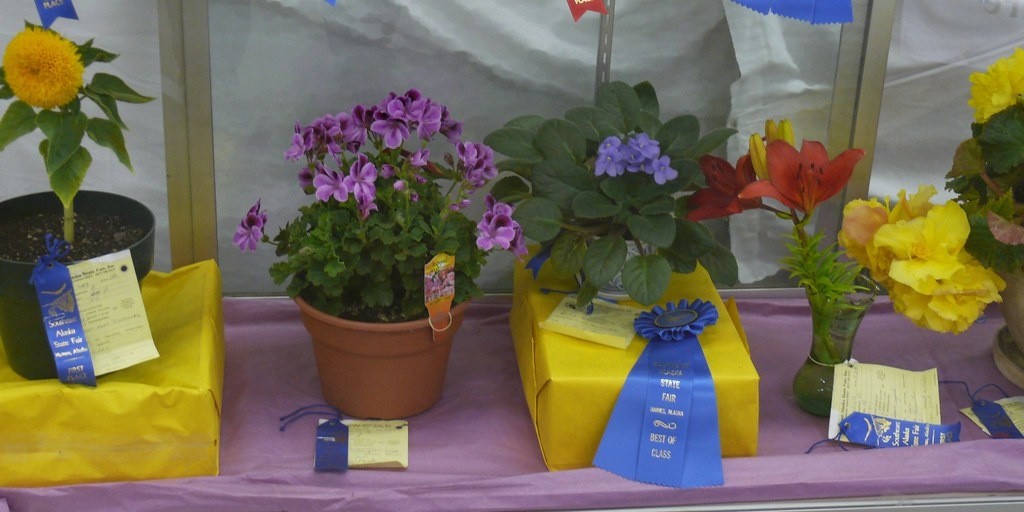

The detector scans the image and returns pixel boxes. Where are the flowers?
[944,45,1024,282]
[687,116,1009,335]
[230,88,530,323]
[0,18,159,243]
[481,80,740,308]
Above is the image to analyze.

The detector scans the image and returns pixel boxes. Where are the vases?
[792,273,877,417]
[292,294,474,420]
[573,234,658,301]
[0,190,156,381]
[992,268,1024,358]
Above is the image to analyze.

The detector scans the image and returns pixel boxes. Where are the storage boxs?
[506,249,760,473]
[0,259,226,491]
